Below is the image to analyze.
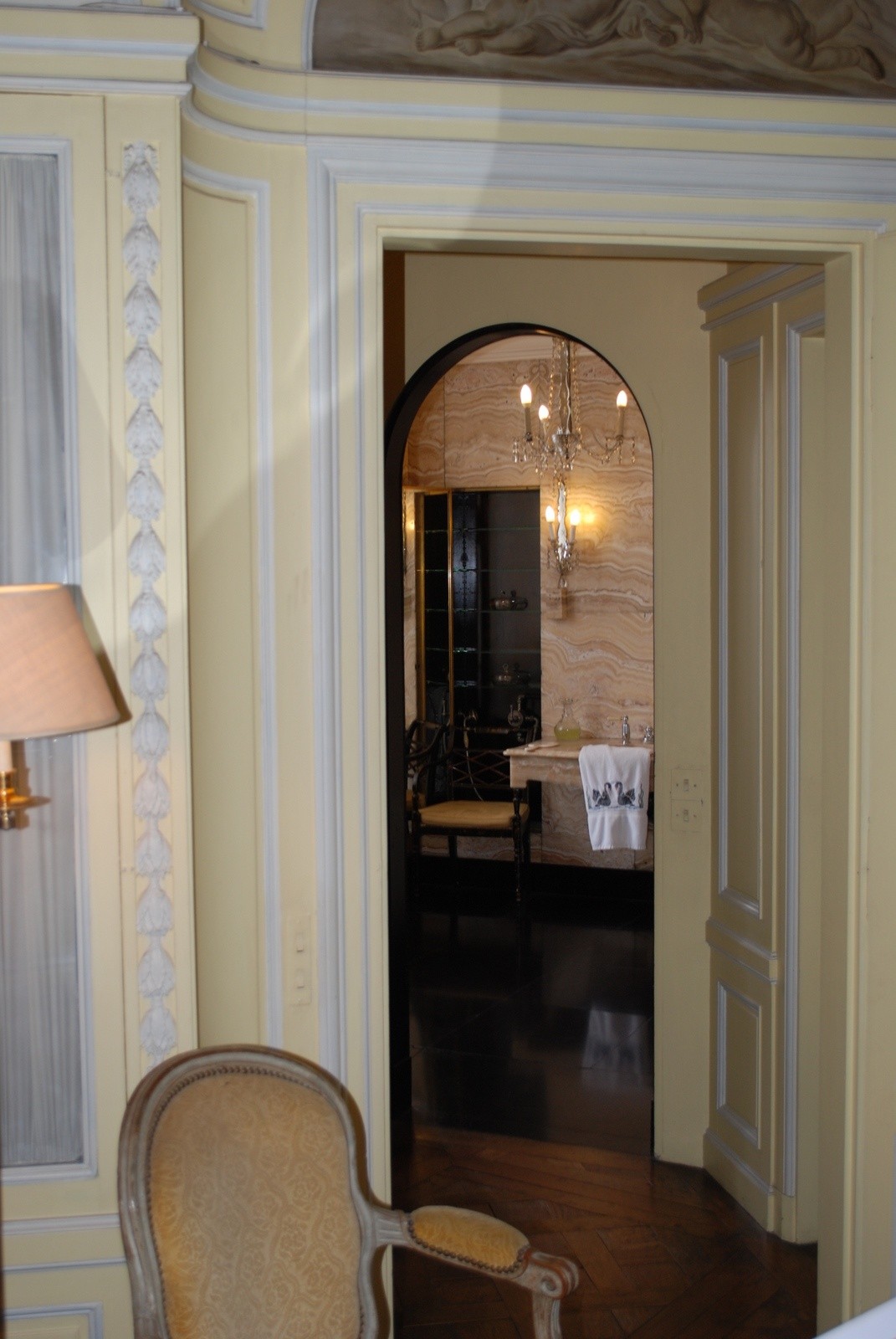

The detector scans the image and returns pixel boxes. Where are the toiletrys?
[554,697,581,741]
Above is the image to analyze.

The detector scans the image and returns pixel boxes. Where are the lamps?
[512,336,638,587]
[0,582,125,830]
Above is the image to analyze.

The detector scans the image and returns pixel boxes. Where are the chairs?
[399,718,539,973]
[115,1043,582,1339]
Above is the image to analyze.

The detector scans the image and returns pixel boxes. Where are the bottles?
[554,698,581,742]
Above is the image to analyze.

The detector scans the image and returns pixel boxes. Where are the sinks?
[557,739,653,753]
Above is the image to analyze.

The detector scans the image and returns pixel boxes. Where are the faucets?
[608,715,631,745]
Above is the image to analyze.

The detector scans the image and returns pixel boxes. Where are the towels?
[578,743,650,852]
